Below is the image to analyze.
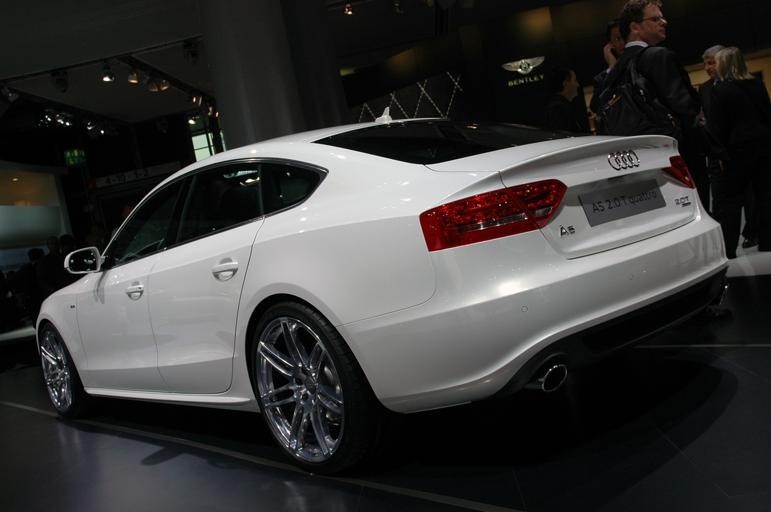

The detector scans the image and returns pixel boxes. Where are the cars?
[36,106,731,467]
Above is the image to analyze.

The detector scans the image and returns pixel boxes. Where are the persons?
[697,46,771,253]
[591,1,733,322]
[1,231,106,330]
[534,58,595,136]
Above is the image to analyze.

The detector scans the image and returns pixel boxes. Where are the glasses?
[637,15,665,24]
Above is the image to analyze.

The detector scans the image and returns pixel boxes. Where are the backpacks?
[594,45,695,163]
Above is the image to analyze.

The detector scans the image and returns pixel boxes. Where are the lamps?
[84,63,203,130]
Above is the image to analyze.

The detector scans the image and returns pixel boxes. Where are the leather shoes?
[742,238,770,252]
